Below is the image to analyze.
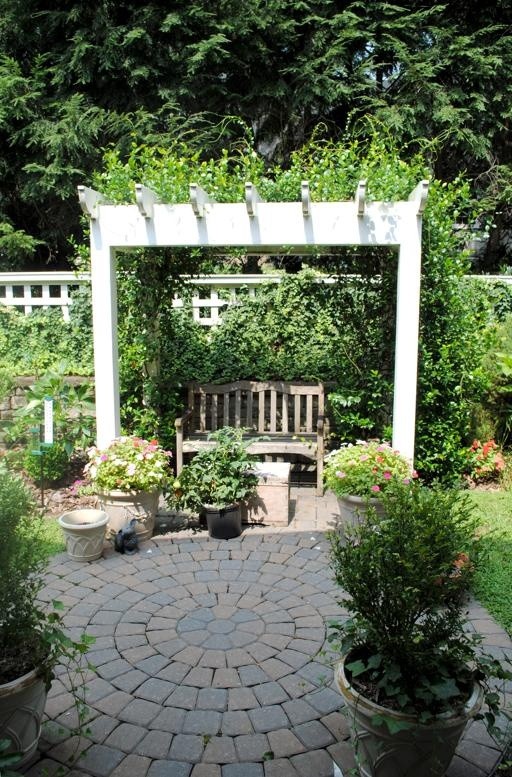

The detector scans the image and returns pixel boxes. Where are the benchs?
[174,379,325,497]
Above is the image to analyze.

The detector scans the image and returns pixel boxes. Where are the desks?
[189,460,291,527]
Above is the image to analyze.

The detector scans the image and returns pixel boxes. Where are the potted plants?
[162,426,272,539]
[298,477,511,777]
[0,446,100,777]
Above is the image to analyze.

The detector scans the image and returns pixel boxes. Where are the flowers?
[319,438,418,505]
[61,435,181,500]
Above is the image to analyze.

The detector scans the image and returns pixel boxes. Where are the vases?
[58,508,110,562]
[97,488,158,542]
[333,491,397,533]
